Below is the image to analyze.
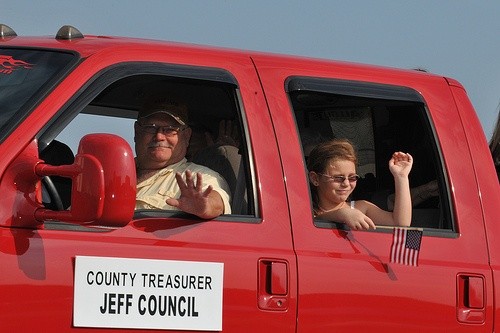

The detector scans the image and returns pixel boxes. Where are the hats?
[138,92,188,126]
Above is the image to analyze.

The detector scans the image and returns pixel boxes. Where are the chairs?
[373,156,441,223]
[193,119,248,215]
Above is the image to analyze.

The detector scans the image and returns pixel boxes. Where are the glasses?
[318,172,360,183]
[137,124,187,135]
